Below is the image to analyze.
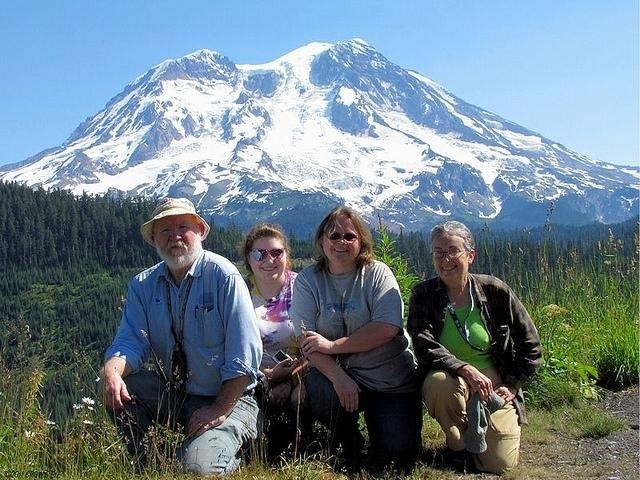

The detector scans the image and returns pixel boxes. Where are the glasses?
[431,248,467,257]
[328,232,358,242]
[249,248,284,261]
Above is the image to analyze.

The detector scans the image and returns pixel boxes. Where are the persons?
[99,197,265,476]
[408,221,545,475]
[243,226,298,457]
[290,205,420,474]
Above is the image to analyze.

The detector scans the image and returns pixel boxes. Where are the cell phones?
[272,349,294,368]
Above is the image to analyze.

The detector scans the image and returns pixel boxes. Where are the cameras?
[169,348,188,384]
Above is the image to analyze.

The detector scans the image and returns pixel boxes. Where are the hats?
[140,198,210,247]
